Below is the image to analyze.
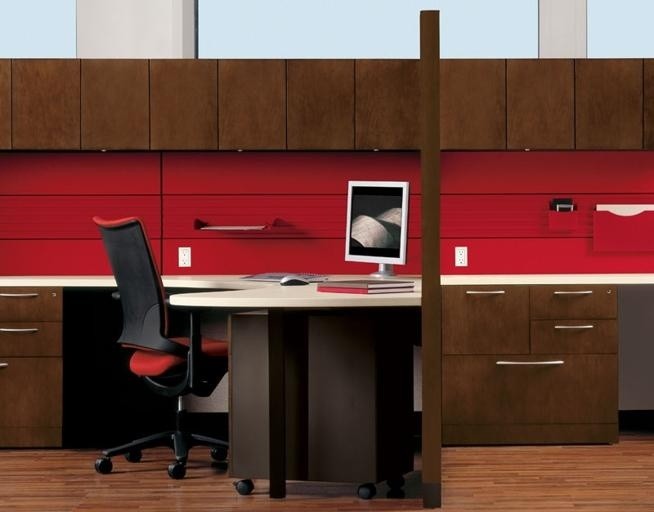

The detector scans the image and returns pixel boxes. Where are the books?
[316,277,415,294]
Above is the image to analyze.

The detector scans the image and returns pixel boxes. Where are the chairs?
[90,214,231,479]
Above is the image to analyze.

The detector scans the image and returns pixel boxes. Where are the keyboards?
[241,272,328,282]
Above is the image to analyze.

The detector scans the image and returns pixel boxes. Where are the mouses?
[280,276,308,286]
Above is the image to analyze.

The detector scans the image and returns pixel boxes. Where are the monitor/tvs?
[345,181,410,277]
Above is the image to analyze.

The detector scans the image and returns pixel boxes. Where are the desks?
[70,275,422,502]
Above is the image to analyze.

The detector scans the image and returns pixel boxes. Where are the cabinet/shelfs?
[440,283,618,448]
[286,59,422,152]
[11,58,149,153]
[575,60,654,153]
[0,287,68,451]
[150,58,287,153]
[1,58,11,153]
[439,59,575,153]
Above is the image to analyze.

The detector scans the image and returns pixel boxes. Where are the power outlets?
[454,246,467,267]
